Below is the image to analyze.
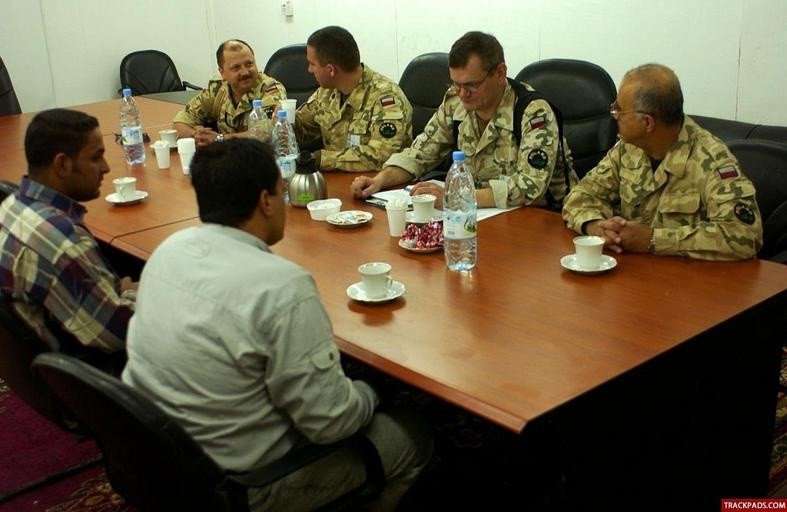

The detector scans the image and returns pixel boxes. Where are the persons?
[173,39,287,148]
[0,108,137,372]
[120,137,430,512]
[350,32,580,210]
[562,63,763,259]
[293,26,414,172]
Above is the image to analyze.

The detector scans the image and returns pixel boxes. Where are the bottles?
[247,100,269,143]
[271,109,297,205]
[119,89,146,165]
[442,151,478,272]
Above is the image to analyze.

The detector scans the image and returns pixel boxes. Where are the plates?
[327,210,373,228]
[151,141,179,150]
[105,191,148,205]
[398,238,443,253]
[346,279,405,303]
[406,211,446,224]
[560,253,617,275]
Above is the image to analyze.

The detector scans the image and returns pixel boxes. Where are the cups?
[153,144,170,169]
[160,130,178,146]
[113,177,137,198]
[359,262,392,298]
[386,206,406,237]
[572,236,605,269]
[411,194,437,221]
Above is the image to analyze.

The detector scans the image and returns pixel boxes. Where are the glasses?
[609,100,640,120]
[447,66,493,93]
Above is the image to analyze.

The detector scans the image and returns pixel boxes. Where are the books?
[366,177,523,223]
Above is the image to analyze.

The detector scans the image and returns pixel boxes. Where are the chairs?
[0,289,102,501]
[115,50,202,98]
[722,136,786,266]
[262,43,320,113]
[29,351,386,512]
[514,57,620,181]
[392,52,453,144]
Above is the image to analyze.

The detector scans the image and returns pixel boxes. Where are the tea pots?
[289,152,328,208]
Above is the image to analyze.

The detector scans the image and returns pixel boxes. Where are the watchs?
[214,134,224,143]
[648,230,655,255]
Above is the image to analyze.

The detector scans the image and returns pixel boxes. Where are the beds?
[1,96,787,434]
